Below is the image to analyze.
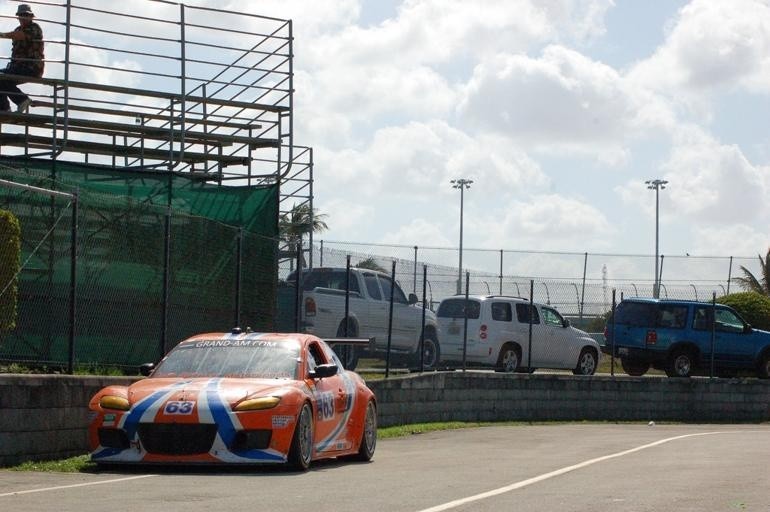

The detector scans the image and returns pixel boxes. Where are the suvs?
[602,298,769,378]
[432,295,604,376]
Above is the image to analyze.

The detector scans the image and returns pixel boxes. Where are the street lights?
[449,178,474,295]
[646,177,669,298]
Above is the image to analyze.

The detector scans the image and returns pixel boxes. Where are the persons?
[0,3,45,113]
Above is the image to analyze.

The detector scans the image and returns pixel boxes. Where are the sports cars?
[87,326,381,473]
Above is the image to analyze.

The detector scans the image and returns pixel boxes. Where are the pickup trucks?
[280,266,443,373]
